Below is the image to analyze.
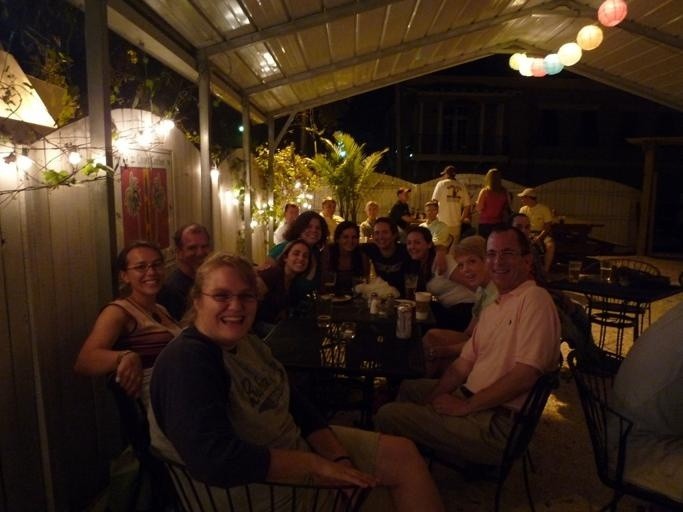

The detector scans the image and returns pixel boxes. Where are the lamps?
[508,0,627,78]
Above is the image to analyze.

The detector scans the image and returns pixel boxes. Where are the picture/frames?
[112,146,180,287]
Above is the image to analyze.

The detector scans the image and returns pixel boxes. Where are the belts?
[494,405,519,421]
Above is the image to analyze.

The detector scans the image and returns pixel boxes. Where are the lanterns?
[509,1,627,77]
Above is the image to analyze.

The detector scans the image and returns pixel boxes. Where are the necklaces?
[127,296,161,325]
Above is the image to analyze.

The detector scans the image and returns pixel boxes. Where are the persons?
[373,224,562,484]
[255,168,555,426]
[73,240,181,414]
[145,251,442,510]
[157,222,211,320]
[602,300,682,510]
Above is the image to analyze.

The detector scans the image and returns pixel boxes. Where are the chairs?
[288,246,682,357]
[416,371,558,510]
[108,374,172,510]
[565,350,682,509]
[149,383,375,510]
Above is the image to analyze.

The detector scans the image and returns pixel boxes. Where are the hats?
[441,166,456,175]
[397,186,410,192]
[518,188,536,198]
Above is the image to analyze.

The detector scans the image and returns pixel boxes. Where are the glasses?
[134,261,164,271]
[212,290,257,302]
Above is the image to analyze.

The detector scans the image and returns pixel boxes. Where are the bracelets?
[331,455,353,464]
[115,350,133,365]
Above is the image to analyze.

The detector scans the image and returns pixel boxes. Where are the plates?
[321,294,351,304]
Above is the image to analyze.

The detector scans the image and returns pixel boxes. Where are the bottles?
[368,290,432,342]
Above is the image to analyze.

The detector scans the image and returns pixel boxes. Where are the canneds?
[394,303,415,340]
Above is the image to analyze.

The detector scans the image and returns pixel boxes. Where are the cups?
[566,260,583,284]
[321,271,336,290]
[599,257,612,284]
[340,320,355,340]
[317,315,330,328]
